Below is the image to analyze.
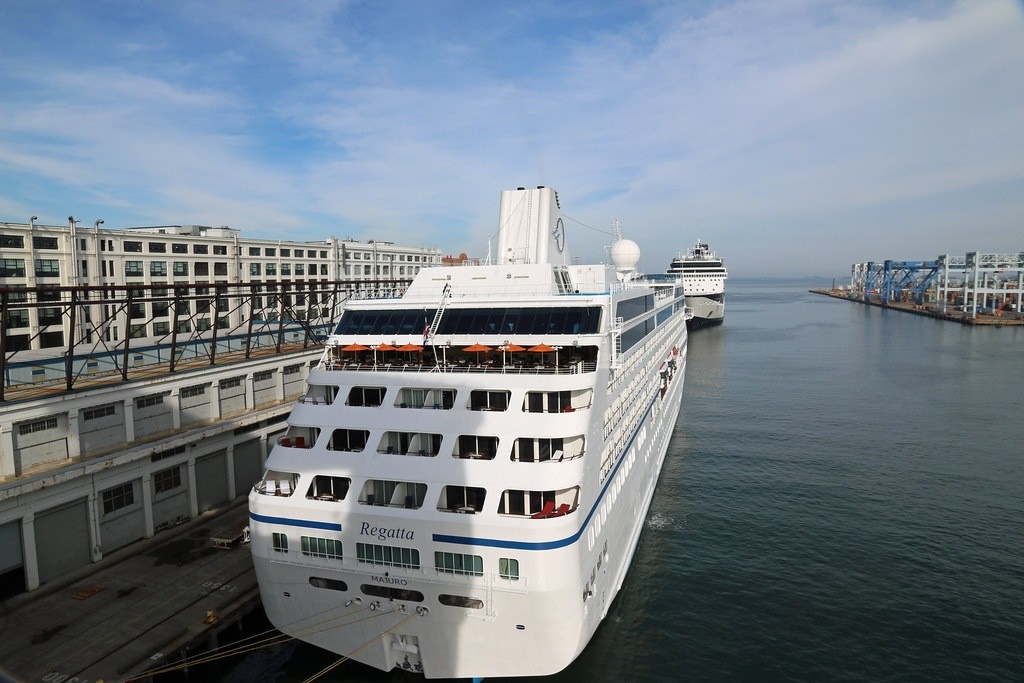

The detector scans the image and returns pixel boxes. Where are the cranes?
[849,251,1024,318]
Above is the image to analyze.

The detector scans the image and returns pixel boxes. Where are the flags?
[424,324,431,341]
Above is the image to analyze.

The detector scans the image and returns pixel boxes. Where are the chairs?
[400,403,406,408]
[530,501,554,519]
[434,404,439,409]
[280,439,291,447]
[542,450,563,462]
[291,437,304,448]
[367,495,375,506]
[403,496,413,509]
[280,479,290,497]
[419,450,428,456]
[560,404,575,412]
[266,480,276,496]
[552,504,570,516]
[387,446,394,453]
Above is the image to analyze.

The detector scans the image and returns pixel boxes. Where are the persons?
[443,283,451,298]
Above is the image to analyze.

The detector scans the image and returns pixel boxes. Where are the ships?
[247,183,690,679]
[666,239,728,327]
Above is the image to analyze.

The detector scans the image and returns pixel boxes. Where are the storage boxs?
[902,284,1023,312]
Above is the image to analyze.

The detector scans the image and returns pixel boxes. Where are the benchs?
[210,520,248,549]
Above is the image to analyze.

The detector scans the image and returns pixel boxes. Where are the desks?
[457,507,475,513]
[469,454,482,459]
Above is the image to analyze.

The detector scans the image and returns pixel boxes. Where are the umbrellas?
[396,342,424,361]
[462,343,493,364]
[340,343,372,361]
[497,343,527,365]
[369,342,397,362]
[527,343,556,366]
